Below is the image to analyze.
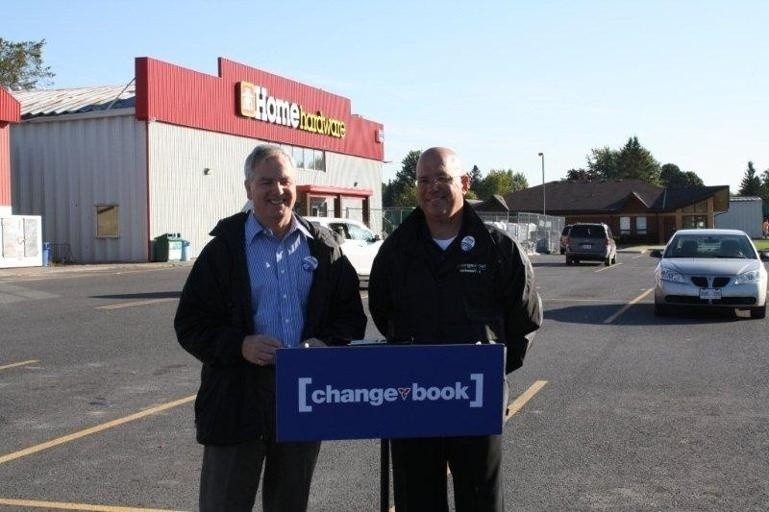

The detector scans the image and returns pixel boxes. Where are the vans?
[559,225,572,254]
[530,229,567,255]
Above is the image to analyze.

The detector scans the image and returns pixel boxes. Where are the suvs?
[302,216,385,283]
[565,222,617,266]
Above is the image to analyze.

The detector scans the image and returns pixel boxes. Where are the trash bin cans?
[42,242,50,267]
[154,233,191,262]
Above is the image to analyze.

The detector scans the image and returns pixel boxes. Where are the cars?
[648,228,768,319]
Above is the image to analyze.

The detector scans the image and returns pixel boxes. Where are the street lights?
[537,153,546,216]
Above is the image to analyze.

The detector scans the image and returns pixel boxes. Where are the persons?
[171,142,370,511]
[362,145,544,511]
